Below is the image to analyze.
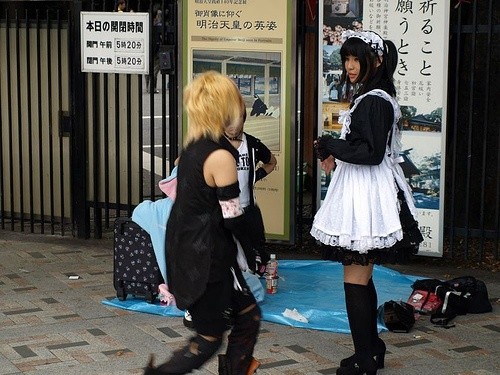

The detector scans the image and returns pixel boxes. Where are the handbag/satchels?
[377,274,492,334]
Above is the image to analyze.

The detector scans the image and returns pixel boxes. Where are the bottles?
[266,254,279,276]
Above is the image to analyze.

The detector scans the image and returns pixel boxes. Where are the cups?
[266,277,278,294]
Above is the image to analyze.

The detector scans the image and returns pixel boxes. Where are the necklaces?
[223,130,244,142]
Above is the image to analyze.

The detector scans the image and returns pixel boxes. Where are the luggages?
[113,216,164,303]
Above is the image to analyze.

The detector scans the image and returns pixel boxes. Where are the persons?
[143,2,174,95]
[222,93,277,273]
[323,71,360,103]
[111,0,126,12]
[143,68,273,375]
[309,29,425,375]
[250,93,265,116]
[129,8,135,12]
[323,19,363,45]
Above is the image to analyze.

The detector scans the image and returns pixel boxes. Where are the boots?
[336,282,385,375]
